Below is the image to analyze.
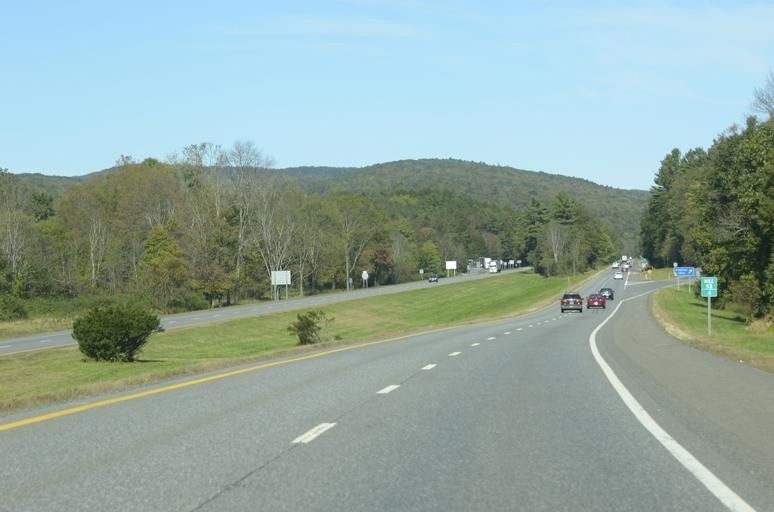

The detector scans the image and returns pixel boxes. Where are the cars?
[612,255,632,281]
[428,275,439,283]
[560,286,616,313]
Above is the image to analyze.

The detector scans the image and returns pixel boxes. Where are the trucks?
[488,262,502,273]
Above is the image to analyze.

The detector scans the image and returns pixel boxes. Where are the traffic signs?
[673,266,695,277]
[701,276,718,297]
